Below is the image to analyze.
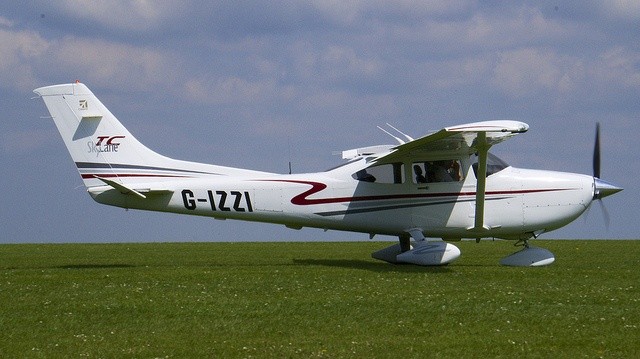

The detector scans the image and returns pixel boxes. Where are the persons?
[430,159,463,183]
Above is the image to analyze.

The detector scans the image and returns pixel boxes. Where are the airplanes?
[31,80,624,268]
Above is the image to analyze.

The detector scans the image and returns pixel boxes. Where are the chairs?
[413,164,425,183]
